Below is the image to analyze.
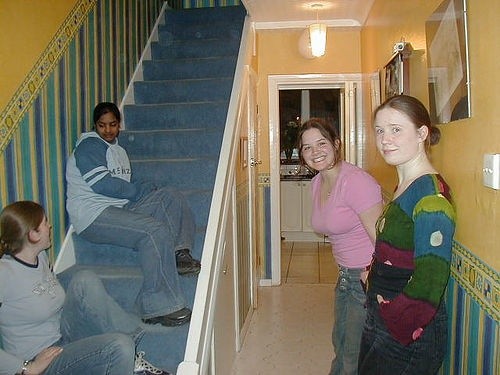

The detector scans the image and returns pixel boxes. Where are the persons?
[66,102,201,327]
[0,202,169,375]
[298,117,386,375]
[357,94,457,375]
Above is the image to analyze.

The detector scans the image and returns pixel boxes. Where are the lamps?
[309,4,326,56]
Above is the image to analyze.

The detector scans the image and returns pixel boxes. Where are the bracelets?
[22,360,29,375]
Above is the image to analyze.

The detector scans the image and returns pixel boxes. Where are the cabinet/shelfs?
[281,180,324,242]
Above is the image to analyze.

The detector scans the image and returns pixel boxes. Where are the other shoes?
[141,306,192,328]
[174,248,203,278]
[132,350,172,375]
